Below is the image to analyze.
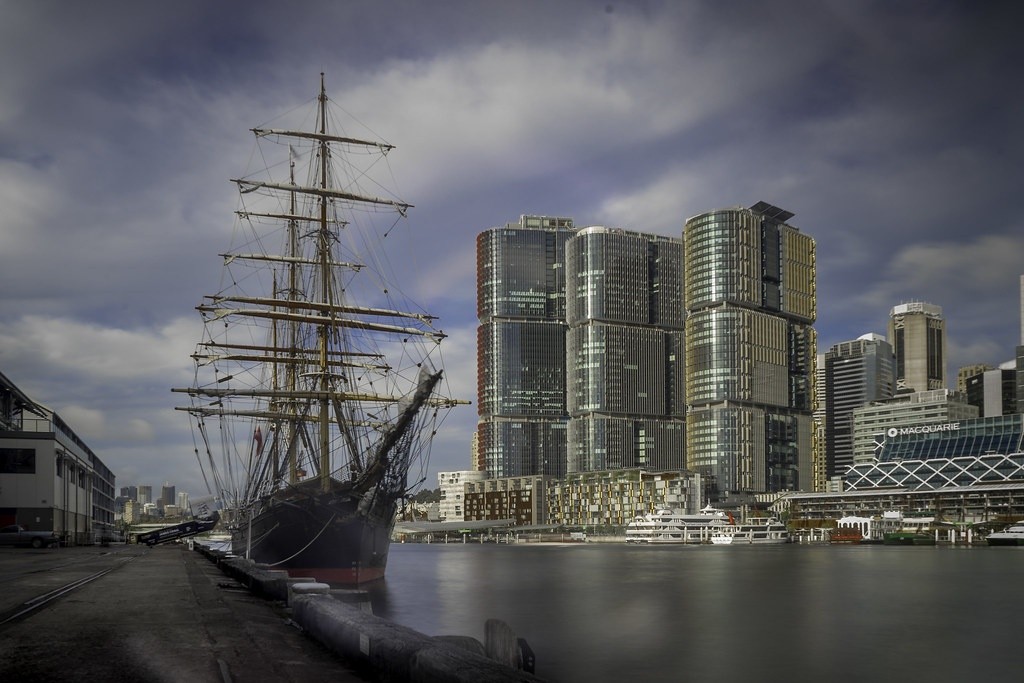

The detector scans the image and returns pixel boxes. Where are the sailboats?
[170,72,472,590]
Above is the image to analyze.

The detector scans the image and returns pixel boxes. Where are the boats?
[985,520,1024,546]
[625,505,738,545]
[710,517,790,544]
